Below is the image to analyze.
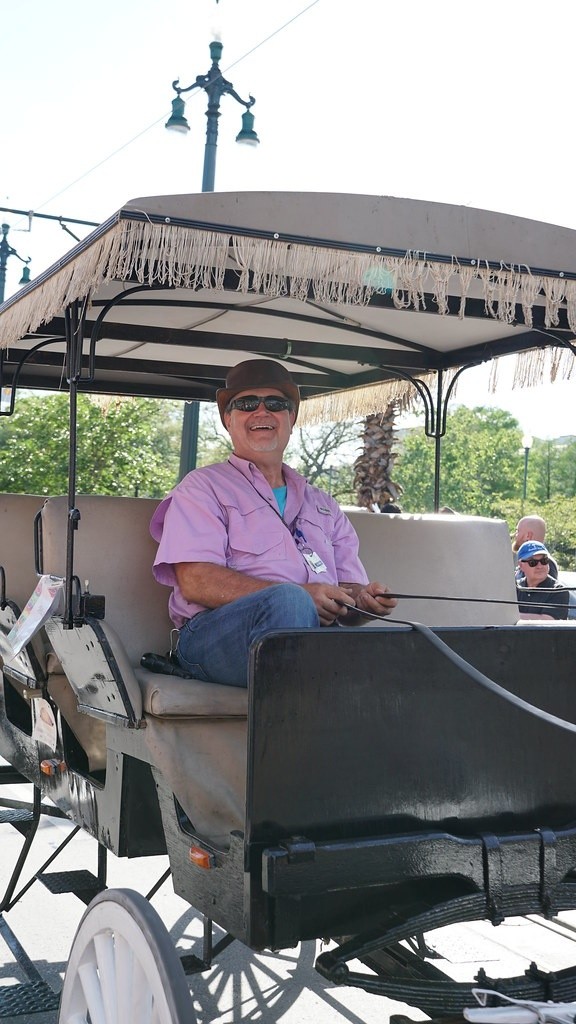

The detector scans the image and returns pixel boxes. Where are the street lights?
[164,41,259,487]
[521,435,533,499]
[0,223,31,306]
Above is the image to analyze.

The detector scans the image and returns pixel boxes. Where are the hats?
[518,540,548,560]
[216,359,300,431]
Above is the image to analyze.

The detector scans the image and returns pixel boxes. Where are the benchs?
[33,496,524,723]
[0,493,66,691]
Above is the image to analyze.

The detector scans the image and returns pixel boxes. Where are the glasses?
[226,395,289,411]
[521,558,549,567]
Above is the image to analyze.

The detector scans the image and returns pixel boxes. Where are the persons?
[380,504,401,514]
[148,359,397,690]
[509,515,569,621]
